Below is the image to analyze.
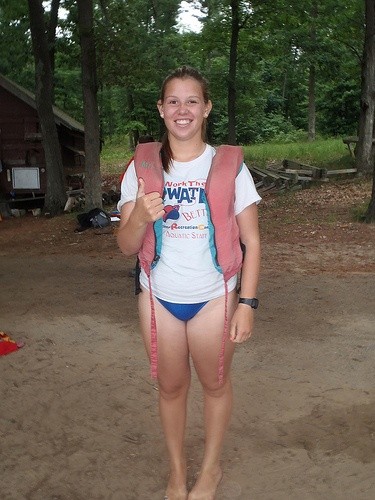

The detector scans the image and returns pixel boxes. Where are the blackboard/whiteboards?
[12,167,41,190]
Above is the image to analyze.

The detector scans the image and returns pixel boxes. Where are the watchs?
[238,297,259,309]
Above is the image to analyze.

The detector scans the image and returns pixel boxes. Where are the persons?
[117,66,262,500]
